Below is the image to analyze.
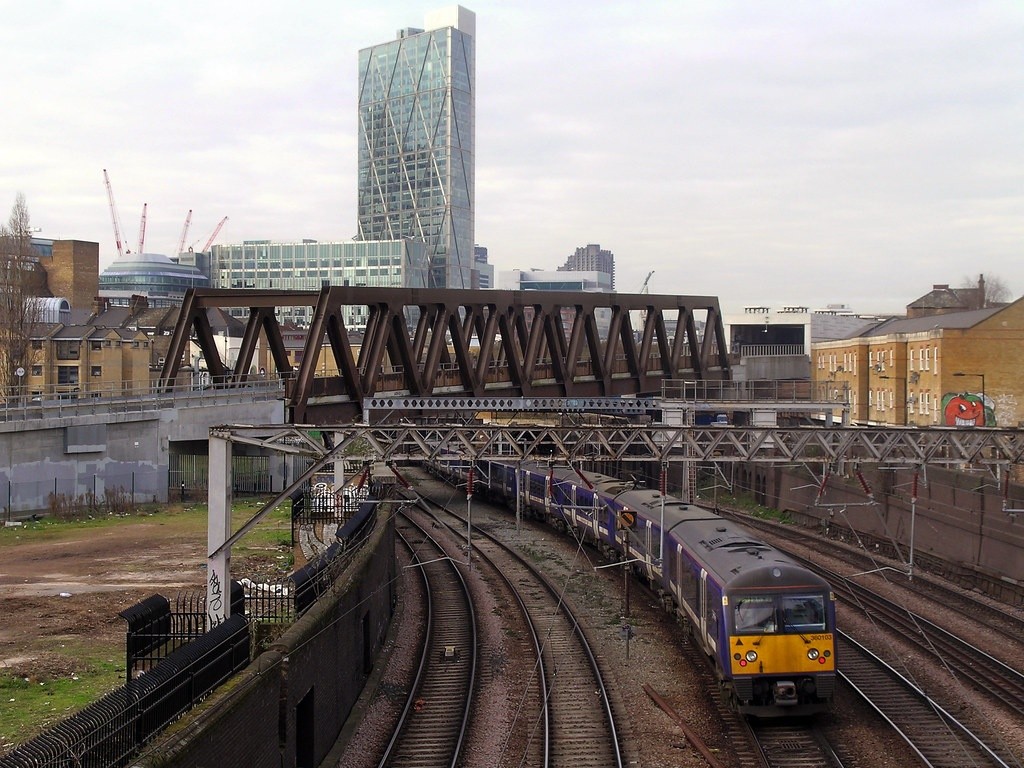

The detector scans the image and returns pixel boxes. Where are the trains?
[423,453,839,719]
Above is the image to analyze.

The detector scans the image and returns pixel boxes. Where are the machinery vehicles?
[102,166,229,259]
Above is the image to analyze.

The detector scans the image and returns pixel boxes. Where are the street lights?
[879,375,908,426]
[952,373,986,426]
